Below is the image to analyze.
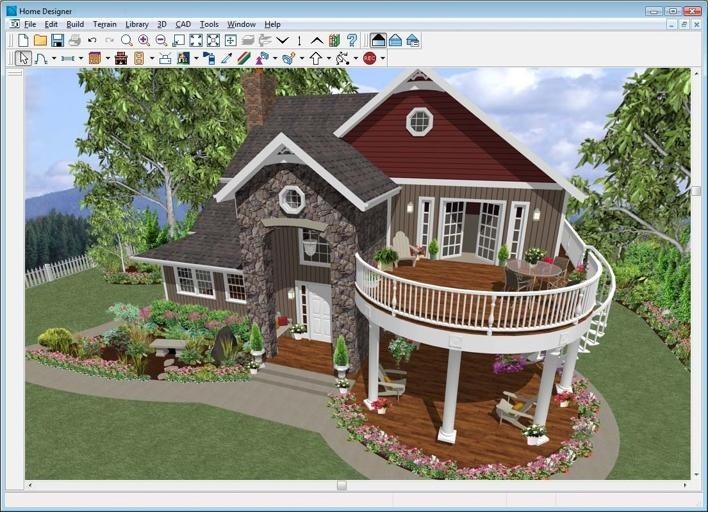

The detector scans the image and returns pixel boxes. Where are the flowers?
[336,379,350,388]
[569,264,587,281]
[289,324,306,334]
[524,248,548,264]
[247,361,259,369]
[554,390,576,401]
[388,338,415,359]
[371,398,392,409]
[493,354,526,374]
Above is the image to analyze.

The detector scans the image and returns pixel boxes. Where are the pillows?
[384,376,393,390]
[509,402,523,415]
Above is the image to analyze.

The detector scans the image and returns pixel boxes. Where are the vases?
[250,369,257,374]
[340,388,347,394]
[560,400,569,407]
[292,334,302,340]
[378,406,386,414]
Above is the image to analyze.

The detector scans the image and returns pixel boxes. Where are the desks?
[149,339,188,357]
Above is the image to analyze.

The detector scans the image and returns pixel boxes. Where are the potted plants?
[333,335,349,379]
[249,322,266,362]
[497,244,509,266]
[373,248,397,272]
[428,239,439,260]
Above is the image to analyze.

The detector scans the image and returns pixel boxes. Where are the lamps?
[407,201,414,214]
[533,208,541,221]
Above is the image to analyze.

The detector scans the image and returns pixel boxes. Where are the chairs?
[390,231,419,268]
[378,364,407,401]
[504,256,580,292]
[495,391,537,430]
